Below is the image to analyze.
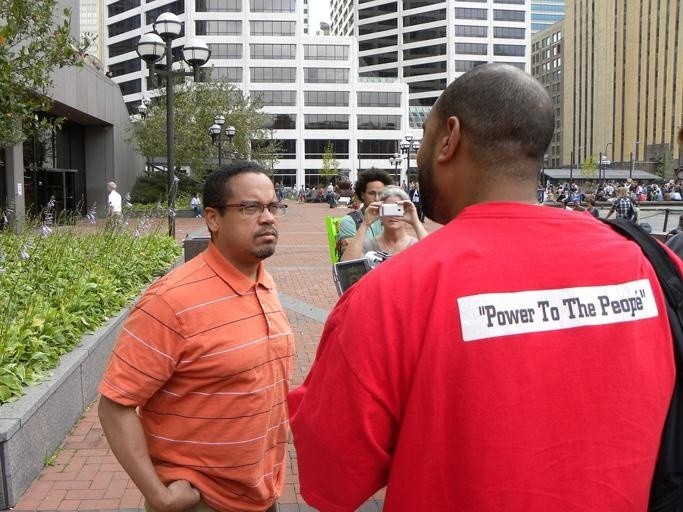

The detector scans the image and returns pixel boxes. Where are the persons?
[534,180,682,220]
[336,185,430,273]
[272,180,340,210]
[396,181,427,221]
[103,180,121,236]
[95,159,298,511]
[341,267,361,290]
[337,167,393,253]
[282,61,681,510]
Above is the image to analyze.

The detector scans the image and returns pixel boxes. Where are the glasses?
[208,200,288,219]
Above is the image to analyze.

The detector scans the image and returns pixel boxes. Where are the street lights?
[399,133,420,191]
[138,95,159,176]
[597,155,610,181]
[631,140,640,178]
[207,113,236,168]
[137,8,211,241]
[389,152,401,182]
[540,153,548,188]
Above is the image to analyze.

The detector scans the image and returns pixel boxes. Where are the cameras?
[379,204,404,216]
[331,250,390,295]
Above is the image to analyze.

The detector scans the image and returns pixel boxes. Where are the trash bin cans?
[182,233,211,263]
